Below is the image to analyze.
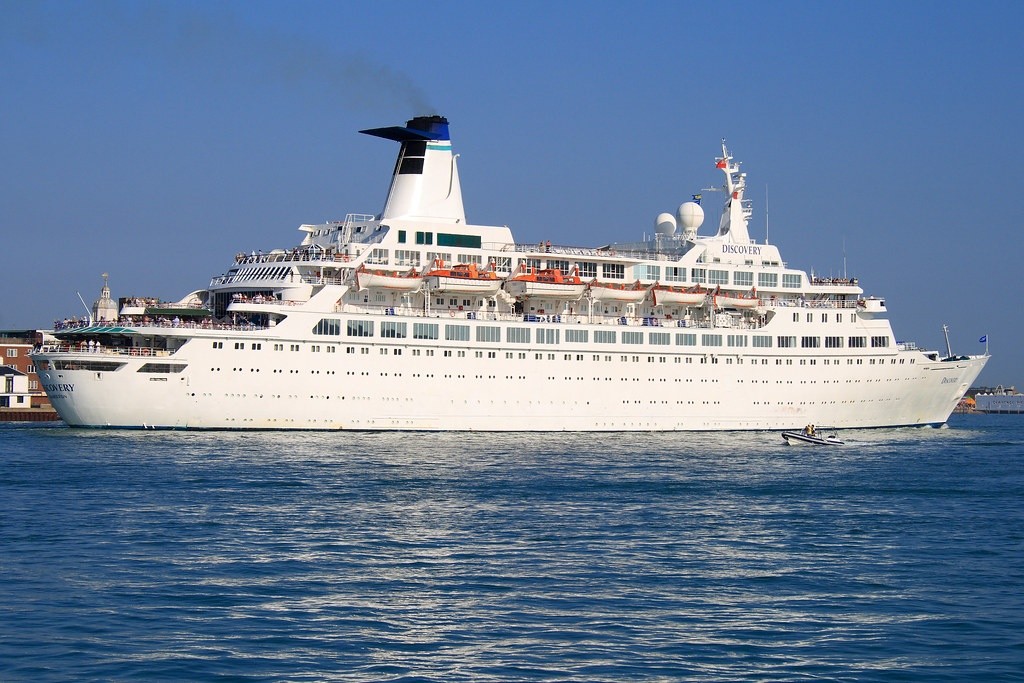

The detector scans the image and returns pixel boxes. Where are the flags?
[980,335,987,343]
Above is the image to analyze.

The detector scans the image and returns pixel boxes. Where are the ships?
[24,114,992,434]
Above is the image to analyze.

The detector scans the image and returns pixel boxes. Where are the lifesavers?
[450,310,456,317]
[288,301,296,306]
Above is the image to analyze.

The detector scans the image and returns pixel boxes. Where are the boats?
[782,431,846,448]
[353,266,760,310]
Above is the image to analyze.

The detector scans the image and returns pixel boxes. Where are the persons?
[33,339,101,353]
[238,292,276,305]
[236,246,312,263]
[54,315,264,331]
[805,423,816,437]
[126,296,173,308]
[539,240,551,253]
[814,276,856,284]
